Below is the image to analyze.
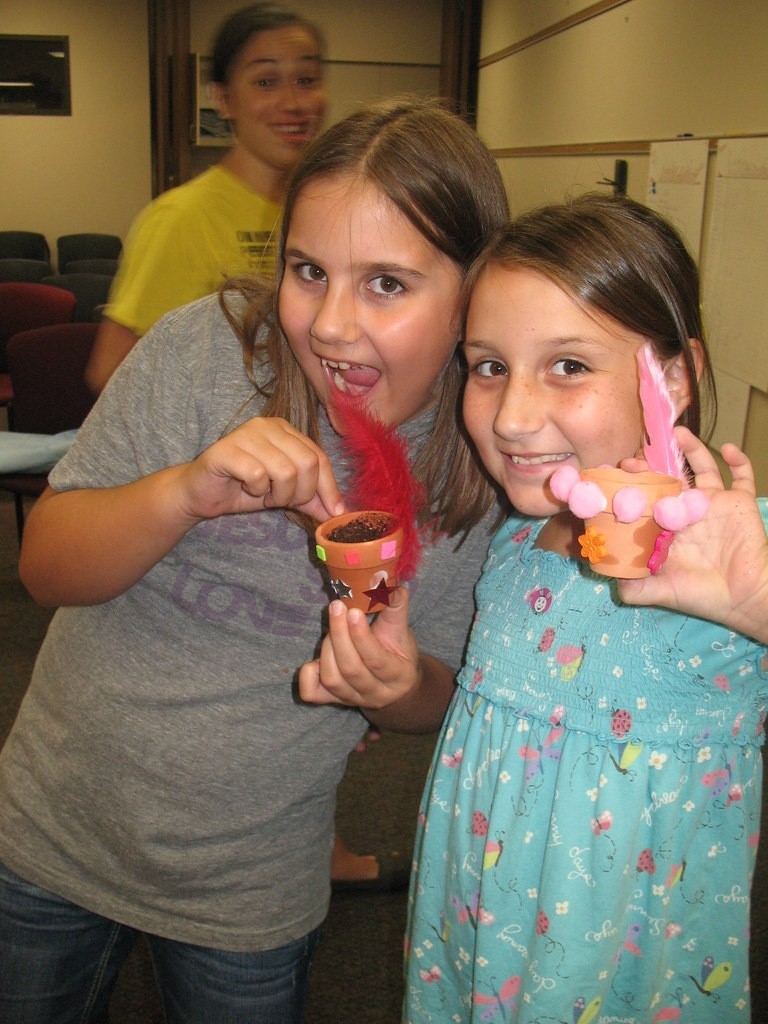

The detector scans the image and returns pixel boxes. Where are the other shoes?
[330,854,411,892]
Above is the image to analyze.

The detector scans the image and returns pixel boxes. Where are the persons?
[402,188,768,1024]
[86,4,415,896]
[0,91,509,1024]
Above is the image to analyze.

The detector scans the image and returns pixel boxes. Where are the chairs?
[0,230,125,555]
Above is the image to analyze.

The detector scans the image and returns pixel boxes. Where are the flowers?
[647,531,677,576]
[578,527,607,565]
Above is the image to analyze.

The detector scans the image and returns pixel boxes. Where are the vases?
[580,467,682,579]
[314,509,404,612]
